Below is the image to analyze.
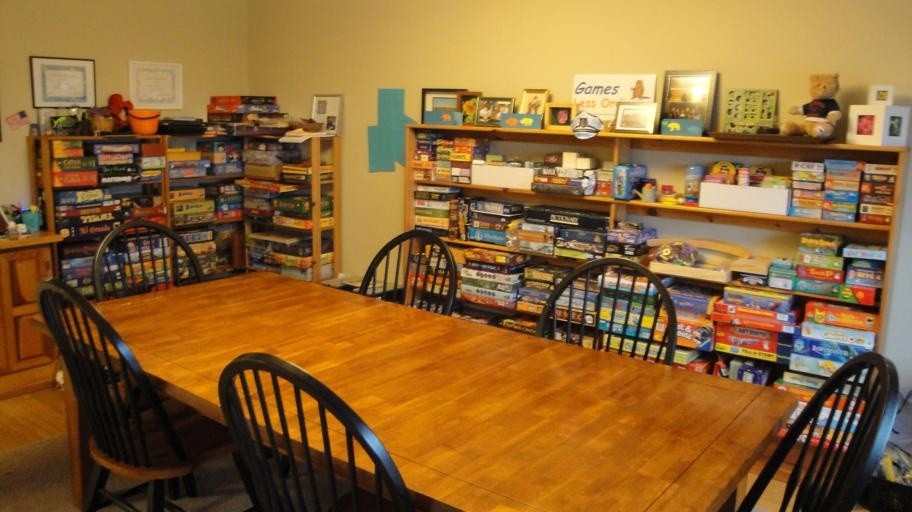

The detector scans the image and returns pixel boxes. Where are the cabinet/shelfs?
[164,113,339,285]
[398,114,912,485]
[27,133,174,300]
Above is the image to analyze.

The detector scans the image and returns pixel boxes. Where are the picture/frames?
[519,88,549,132]
[310,93,344,132]
[32,57,97,110]
[478,98,512,126]
[419,87,467,126]
[544,103,576,132]
[457,91,481,128]
[611,99,661,134]
[659,69,718,129]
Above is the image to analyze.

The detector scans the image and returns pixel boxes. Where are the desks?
[0,229,66,400]
[33,271,799,510]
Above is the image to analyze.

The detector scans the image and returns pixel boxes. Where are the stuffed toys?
[779,72,842,139]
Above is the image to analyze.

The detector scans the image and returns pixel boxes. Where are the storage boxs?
[471,161,542,192]
[695,177,791,217]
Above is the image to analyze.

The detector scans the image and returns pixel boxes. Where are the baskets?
[292,118,323,131]
[89,112,113,133]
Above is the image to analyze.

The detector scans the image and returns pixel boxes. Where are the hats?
[569,110,603,140]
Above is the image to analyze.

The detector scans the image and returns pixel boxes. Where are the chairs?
[355,229,459,317]
[214,352,411,509]
[92,219,209,303]
[22,280,236,511]
[728,349,911,512]
[536,257,677,372]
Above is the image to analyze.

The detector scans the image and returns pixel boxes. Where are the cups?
[21,213,42,234]
